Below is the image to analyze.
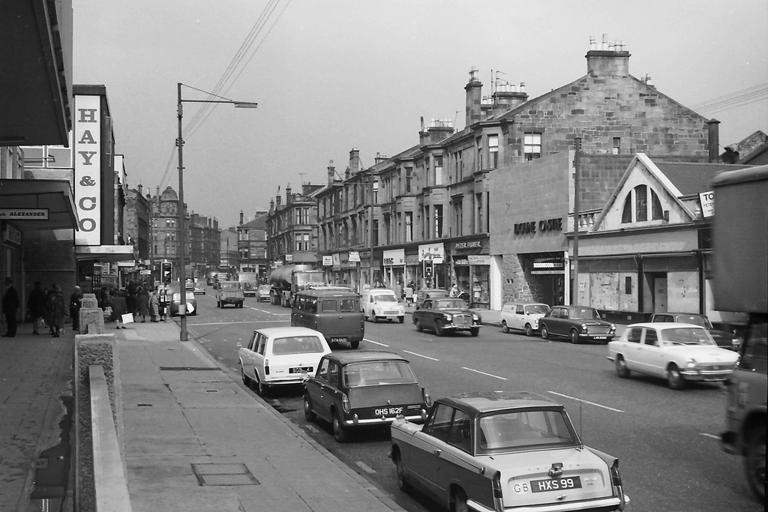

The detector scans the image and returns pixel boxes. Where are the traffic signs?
[174,80,264,341]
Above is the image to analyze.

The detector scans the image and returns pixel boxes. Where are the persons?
[125,279,170,323]
[0,276,83,338]
[401,280,414,303]
[449,284,457,297]
